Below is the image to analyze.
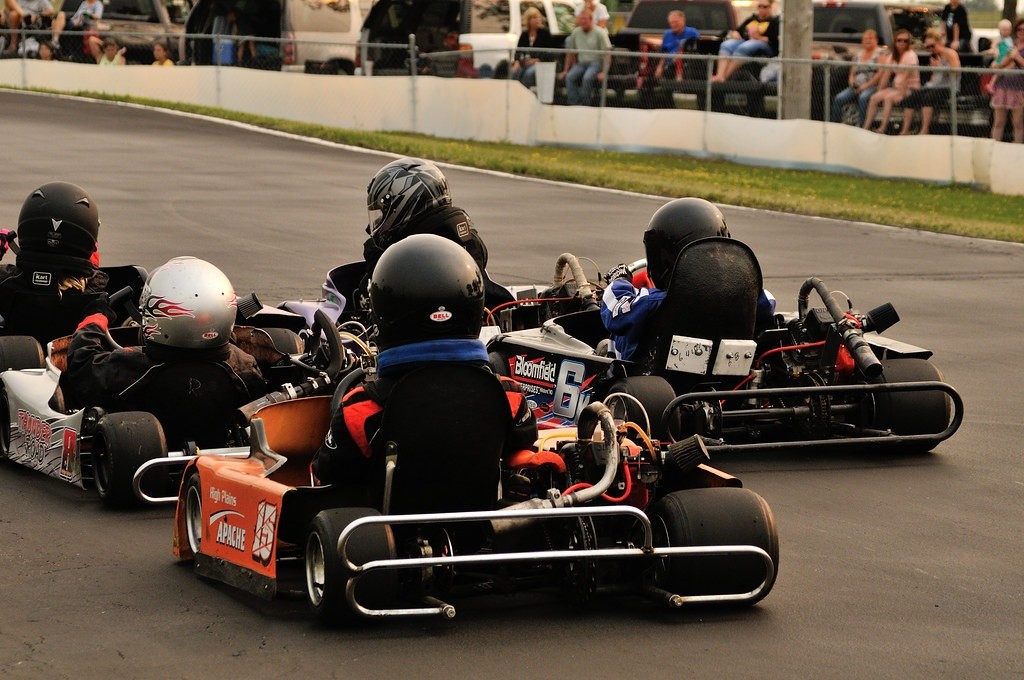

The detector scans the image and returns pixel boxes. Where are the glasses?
[1017,28,1024,32]
[759,5,769,8]
[926,45,934,49]
[897,39,908,43]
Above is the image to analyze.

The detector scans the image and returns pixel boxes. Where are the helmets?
[643,197,731,291]
[139,256,237,364]
[17,181,99,260]
[367,157,453,250]
[370,234,484,352]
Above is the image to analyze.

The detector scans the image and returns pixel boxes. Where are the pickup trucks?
[640,0,946,122]
[354,0,583,81]
[4,0,200,67]
[549,0,779,109]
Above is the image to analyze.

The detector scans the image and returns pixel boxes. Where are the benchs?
[0,1,188,56]
[457,30,993,134]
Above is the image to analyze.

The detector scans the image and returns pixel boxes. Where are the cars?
[832,53,1015,143]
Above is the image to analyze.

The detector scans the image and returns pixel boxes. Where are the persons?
[600,197,776,367]
[0,182,271,450]
[638,10,700,109]
[707,0,780,84]
[0,0,256,68]
[979,19,1024,143]
[940,0,971,52]
[830,21,961,135]
[556,0,613,106]
[510,7,554,88]
[311,157,537,515]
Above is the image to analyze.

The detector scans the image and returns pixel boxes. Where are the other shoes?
[51,41,60,49]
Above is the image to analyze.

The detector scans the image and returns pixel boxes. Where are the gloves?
[0,228,12,251]
[606,263,633,285]
[83,292,117,325]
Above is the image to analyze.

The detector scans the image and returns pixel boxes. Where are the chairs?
[0,287,112,355]
[282,360,506,544]
[134,359,256,451]
[622,235,767,386]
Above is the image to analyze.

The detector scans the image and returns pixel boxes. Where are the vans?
[179,0,381,76]
